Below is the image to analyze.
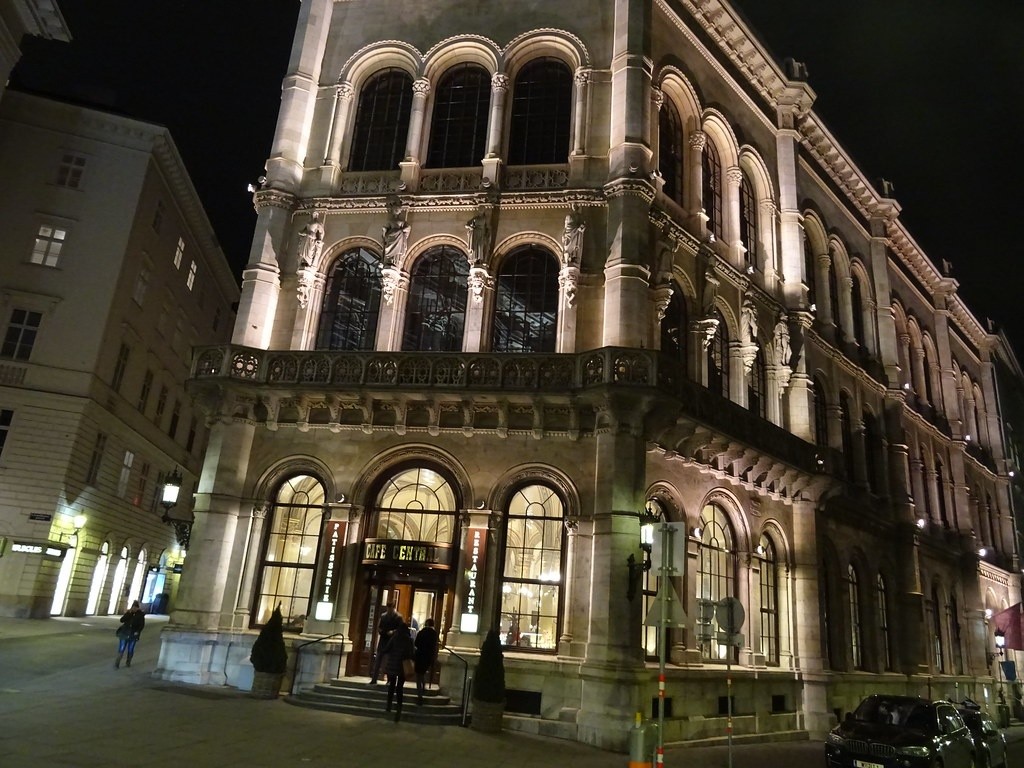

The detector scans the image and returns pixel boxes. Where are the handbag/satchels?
[116,623,132,640]
[403,658,415,678]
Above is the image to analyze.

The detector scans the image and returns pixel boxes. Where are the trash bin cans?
[149,593,169,614]
[626,712,659,768]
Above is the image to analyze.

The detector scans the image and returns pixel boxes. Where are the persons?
[115,600,145,670]
[382,205,412,269]
[465,206,491,265]
[703,257,720,315]
[656,225,679,272]
[741,289,758,343]
[774,312,792,365]
[561,215,585,268]
[296,211,325,268]
[370,603,439,723]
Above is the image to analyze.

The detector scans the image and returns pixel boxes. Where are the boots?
[114,653,123,670]
[126,655,133,667]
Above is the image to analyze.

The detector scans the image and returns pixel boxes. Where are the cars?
[823,694,977,768]
[949,703,1008,768]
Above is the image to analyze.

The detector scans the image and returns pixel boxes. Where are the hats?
[425,619,434,626]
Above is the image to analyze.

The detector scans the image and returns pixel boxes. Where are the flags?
[992,604,1024,651]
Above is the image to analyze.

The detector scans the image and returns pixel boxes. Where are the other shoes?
[395,716,401,722]
[369,679,377,684]
[417,700,423,705]
[386,706,392,712]
[386,681,391,685]
